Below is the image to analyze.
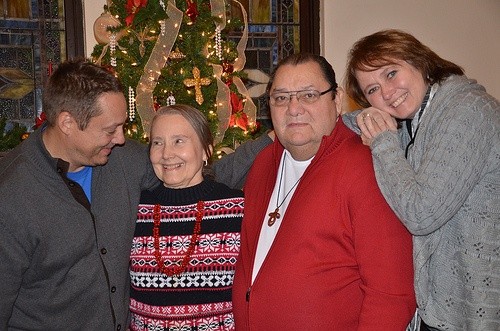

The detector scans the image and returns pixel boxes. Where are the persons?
[337,28,500,331]
[232,54,417,331]
[0,56,274,331]
[125,103,246,331]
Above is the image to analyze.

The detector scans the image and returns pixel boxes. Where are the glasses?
[269,85,336,106]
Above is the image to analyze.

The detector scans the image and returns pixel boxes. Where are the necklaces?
[152,198,207,277]
[267,148,302,228]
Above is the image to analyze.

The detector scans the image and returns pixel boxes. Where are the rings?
[363,113,371,118]
